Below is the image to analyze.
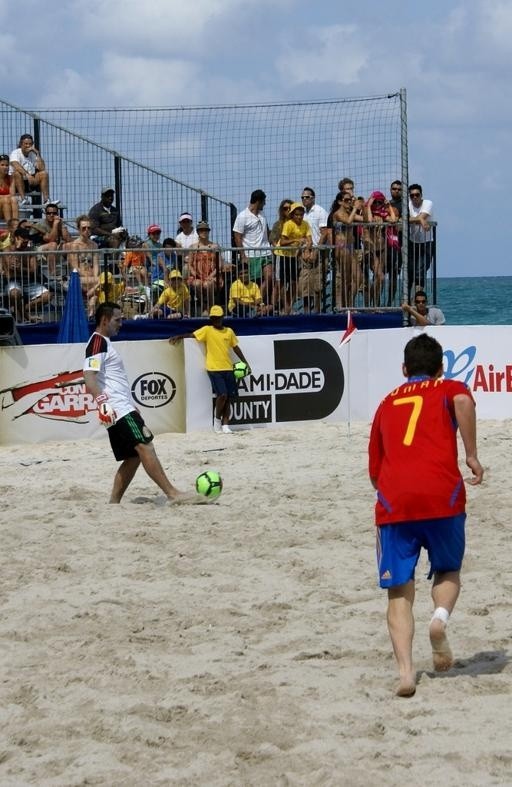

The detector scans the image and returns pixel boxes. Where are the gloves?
[96,393,118,426]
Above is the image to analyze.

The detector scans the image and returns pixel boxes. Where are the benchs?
[0,190,152,313]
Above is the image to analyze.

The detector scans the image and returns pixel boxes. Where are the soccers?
[196,470,222,497]
[233,361,249,378]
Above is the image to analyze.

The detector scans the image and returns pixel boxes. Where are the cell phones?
[358,197,363,200]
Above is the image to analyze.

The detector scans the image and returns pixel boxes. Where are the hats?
[208,305,224,318]
[289,203,304,214]
[98,271,113,285]
[178,214,192,223]
[100,187,114,196]
[168,269,182,280]
[148,224,160,234]
[196,220,210,233]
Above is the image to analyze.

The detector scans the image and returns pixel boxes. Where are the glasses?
[301,195,313,201]
[409,192,421,199]
[342,198,353,203]
[282,207,290,211]
[391,187,403,192]
[46,211,58,216]
[415,299,428,306]
[79,226,91,232]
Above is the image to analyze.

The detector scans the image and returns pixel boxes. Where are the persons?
[1,134,60,222]
[82,302,197,504]
[368,332,484,696]
[168,305,251,433]
[1,178,445,328]
